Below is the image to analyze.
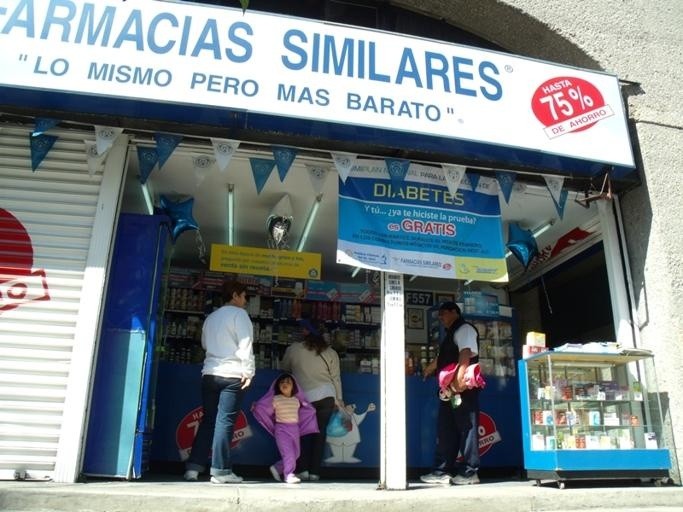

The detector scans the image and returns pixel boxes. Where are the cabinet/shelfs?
[157,251,380,374]
[515,350,671,484]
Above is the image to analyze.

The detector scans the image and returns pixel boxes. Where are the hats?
[296,318,322,336]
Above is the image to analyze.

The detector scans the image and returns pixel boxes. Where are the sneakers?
[185,465,480,485]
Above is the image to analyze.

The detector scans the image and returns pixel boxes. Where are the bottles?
[419,345,436,376]
[163,318,186,338]
[406,352,417,376]
[163,345,191,365]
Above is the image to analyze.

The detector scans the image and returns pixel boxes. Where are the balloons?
[500,212,545,277]
[265,211,296,250]
[159,193,201,247]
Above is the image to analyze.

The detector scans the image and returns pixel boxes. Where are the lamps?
[227,183,234,247]
[296,193,323,252]
[461,219,556,288]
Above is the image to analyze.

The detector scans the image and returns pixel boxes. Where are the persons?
[418,300,484,485]
[182,281,257,486]
[278,317,345,481]
[247,373,321,485]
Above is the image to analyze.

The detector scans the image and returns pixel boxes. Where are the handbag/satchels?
[327,408,351,436]
